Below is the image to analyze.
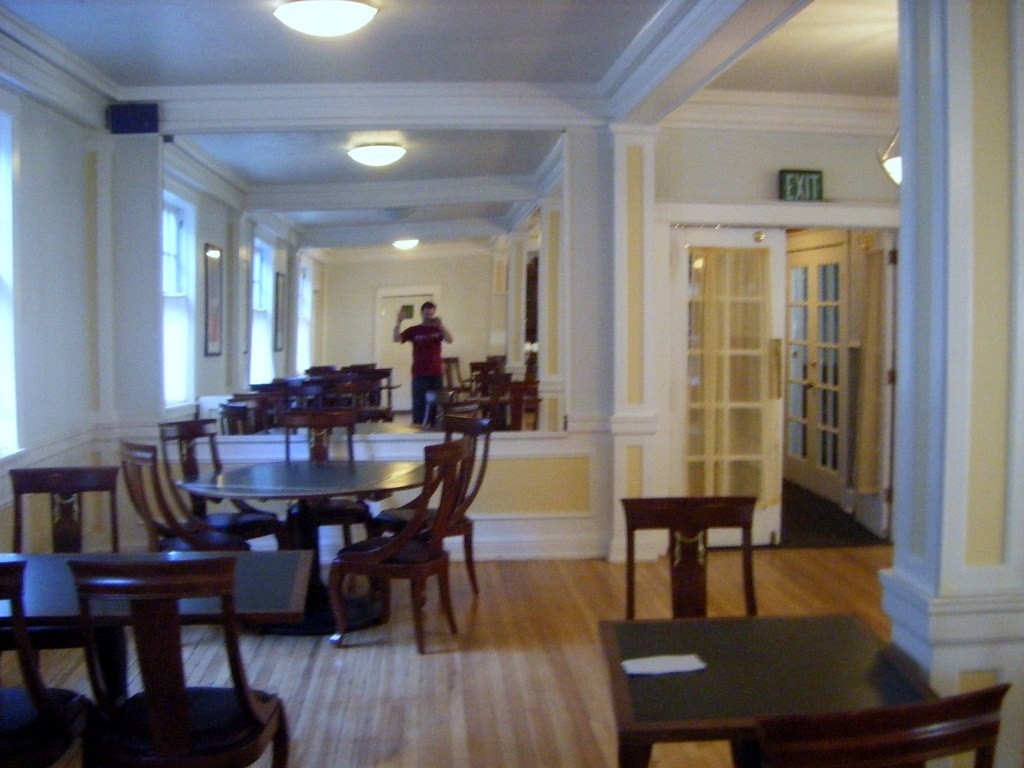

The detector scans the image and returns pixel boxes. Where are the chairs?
[221,352,540,436]
[1,407,493,768]
[621,493,761,768]
[757,680,1011,768]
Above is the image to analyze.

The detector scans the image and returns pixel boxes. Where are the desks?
[175,461,441,638]
[597,610,932,768]
[0,552,312,700]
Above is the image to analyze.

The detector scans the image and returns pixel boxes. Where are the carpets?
[709,477,894,551]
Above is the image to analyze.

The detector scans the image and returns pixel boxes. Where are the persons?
[393,302,453,426]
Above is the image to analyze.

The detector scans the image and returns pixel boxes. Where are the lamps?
[880,127,902,187]
[271,0,382,38]
[346,143,407,168]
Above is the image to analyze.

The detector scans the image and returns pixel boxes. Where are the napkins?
[621,653,706,675]
